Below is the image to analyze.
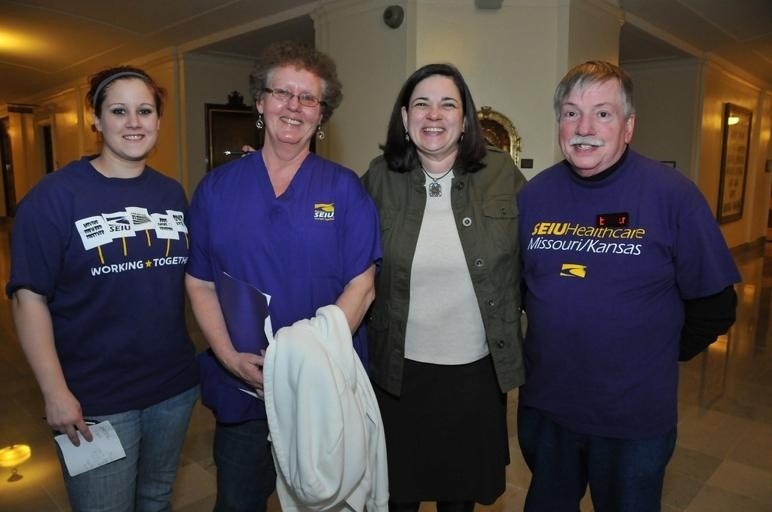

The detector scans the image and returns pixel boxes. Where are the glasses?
[261,88,327,108]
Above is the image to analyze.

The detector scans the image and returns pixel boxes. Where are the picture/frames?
[205,102,317,169]
[717,103,753,225]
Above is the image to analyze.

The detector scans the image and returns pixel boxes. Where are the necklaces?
[421,165,454,197]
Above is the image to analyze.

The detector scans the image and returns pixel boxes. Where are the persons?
[5,63,201,512]
[516,59,743,512]
[358,62,530,512]
[184,39,384,511]
